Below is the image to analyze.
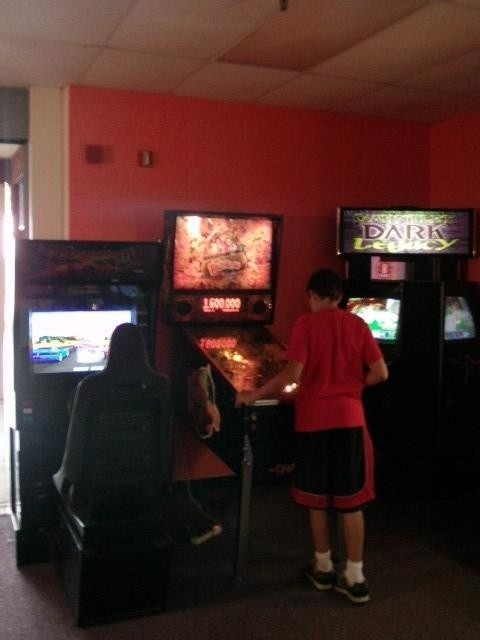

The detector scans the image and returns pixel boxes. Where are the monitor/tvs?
[346,296,401,345]
[191,327,283,401]
[444,296,478,342]
[27,304,137,376]
[173,215,276,293]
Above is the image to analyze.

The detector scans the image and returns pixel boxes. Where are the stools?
[54,495,177,629]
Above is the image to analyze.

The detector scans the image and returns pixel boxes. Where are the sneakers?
[302,556,335,591]
[331,568,371,603]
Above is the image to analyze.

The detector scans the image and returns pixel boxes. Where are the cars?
[32,346,70,364]
[72,338,110,360]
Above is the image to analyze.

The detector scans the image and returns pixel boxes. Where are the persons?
[235,267,389,604]
